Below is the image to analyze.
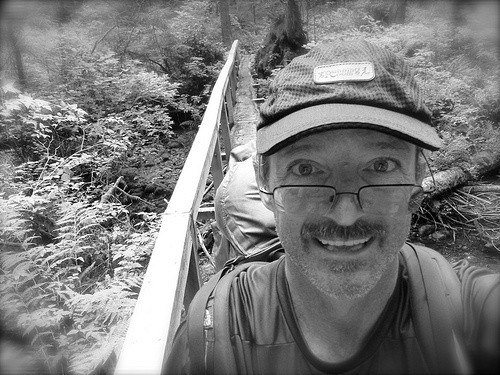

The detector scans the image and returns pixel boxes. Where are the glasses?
[258,148,436,217]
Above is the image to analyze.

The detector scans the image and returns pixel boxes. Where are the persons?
[160,31,500,375]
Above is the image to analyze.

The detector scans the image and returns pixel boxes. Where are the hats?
[255,37,443,152]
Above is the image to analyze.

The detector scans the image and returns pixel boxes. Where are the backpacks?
[185,142,474,375]
